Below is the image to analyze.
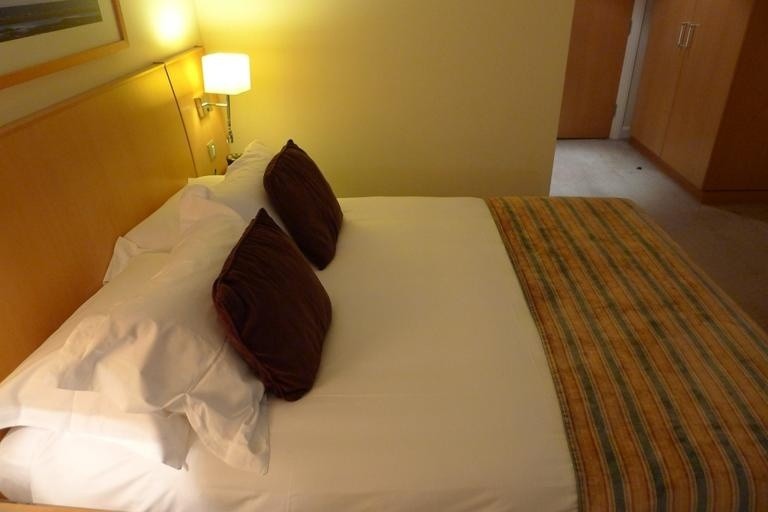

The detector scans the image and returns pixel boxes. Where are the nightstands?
[1,499,109,511]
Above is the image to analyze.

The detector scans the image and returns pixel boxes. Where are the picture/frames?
[0,0,129,90]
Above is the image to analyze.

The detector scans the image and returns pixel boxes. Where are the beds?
[0,46,767,511]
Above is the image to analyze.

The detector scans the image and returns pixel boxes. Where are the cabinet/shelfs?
[629,1,767,202]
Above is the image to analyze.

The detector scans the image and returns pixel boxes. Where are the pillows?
[100,175,225,284]
[58,184,271,477]
[0,237,200,471]
[213,141,292,237]
[212,208,333,402]
[262,139,343,271]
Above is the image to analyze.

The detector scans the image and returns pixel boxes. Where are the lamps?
[195,53,251,144]
